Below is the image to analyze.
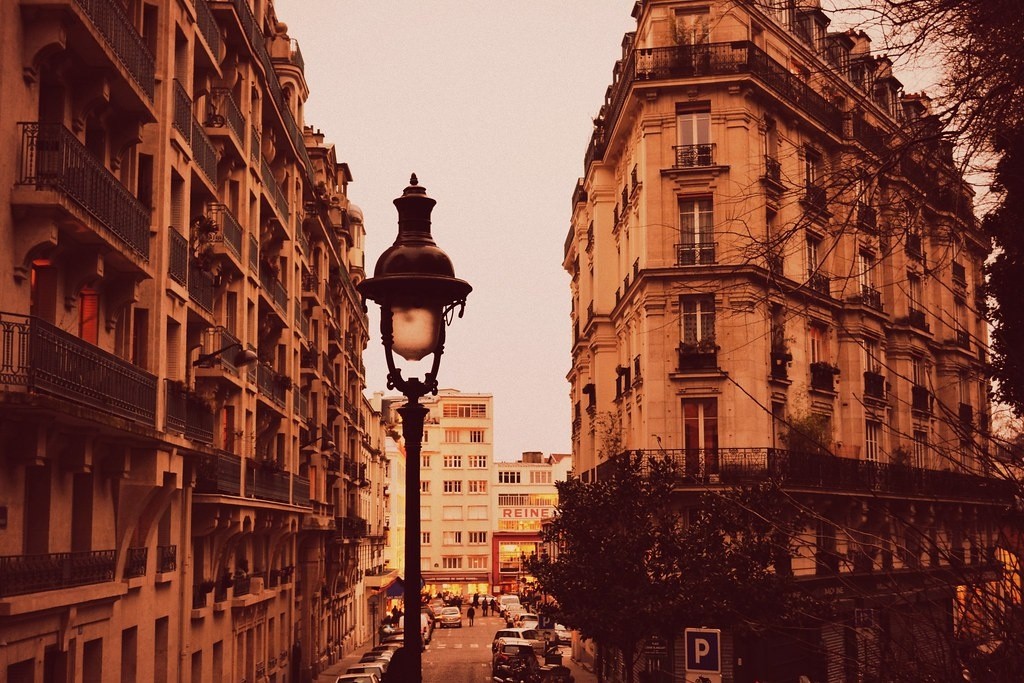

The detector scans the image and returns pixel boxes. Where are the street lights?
[357,169,473,683]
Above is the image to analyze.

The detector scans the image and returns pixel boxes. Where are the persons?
[392,605,404,627]
[467,605,475,627]
[437,591,442,598]
[482,597,488,617]
[450,597,463,614]
[490,598,495,616]
[472,593,480,609]
[506,619,514,628]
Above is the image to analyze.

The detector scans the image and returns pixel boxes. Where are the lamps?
[193,341,259,374]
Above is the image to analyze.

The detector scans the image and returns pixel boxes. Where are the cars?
[335,599,446,683]
[492,595,573,683]
[469,593,497,605]
[440,606,462,628]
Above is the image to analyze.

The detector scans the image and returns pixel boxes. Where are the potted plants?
[911,384,929,411]
[810,362,841,392]
[215,567,232,603]
[193,578,215,609]
[270,568,282,588]
[815,548,842,574]
[848,548,878,572]
[864,365,885,398]
[282,565,294,584]
[673,335,720,369]
[769,336,793,380]
[252,564,268,589]
[234,559,250,597]
